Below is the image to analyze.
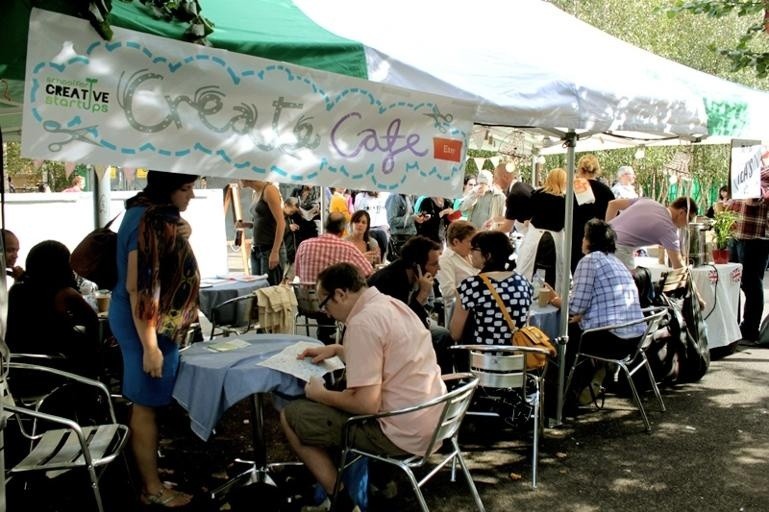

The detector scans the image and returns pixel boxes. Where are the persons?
[107,169,201,507]
[279,260,451,510]
[2,239,103,414]
[0,227,25,343]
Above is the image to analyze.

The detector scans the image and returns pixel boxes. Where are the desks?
[650,264,741,359]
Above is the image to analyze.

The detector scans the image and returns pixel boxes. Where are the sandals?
[139,485,193,508]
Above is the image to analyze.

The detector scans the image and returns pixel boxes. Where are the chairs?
[0,255,558,512]
[631,269,692,395]
[561,305,670,434]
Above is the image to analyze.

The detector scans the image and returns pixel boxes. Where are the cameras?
[419,211,429,219]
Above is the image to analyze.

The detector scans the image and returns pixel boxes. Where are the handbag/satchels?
[68,206,122,292]
[511,325,559,372]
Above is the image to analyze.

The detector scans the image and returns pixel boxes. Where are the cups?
[538,287,550,307]
[96,297,109,312]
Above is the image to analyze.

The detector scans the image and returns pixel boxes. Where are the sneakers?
[319,296,332,313]
[577,365,606,406]
[300,493,364,512]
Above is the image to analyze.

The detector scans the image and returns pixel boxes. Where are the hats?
[477,169,493,185]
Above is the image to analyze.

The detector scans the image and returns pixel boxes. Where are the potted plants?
[707,207,746,265]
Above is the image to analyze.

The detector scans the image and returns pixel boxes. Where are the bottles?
[532,271,545,292]
